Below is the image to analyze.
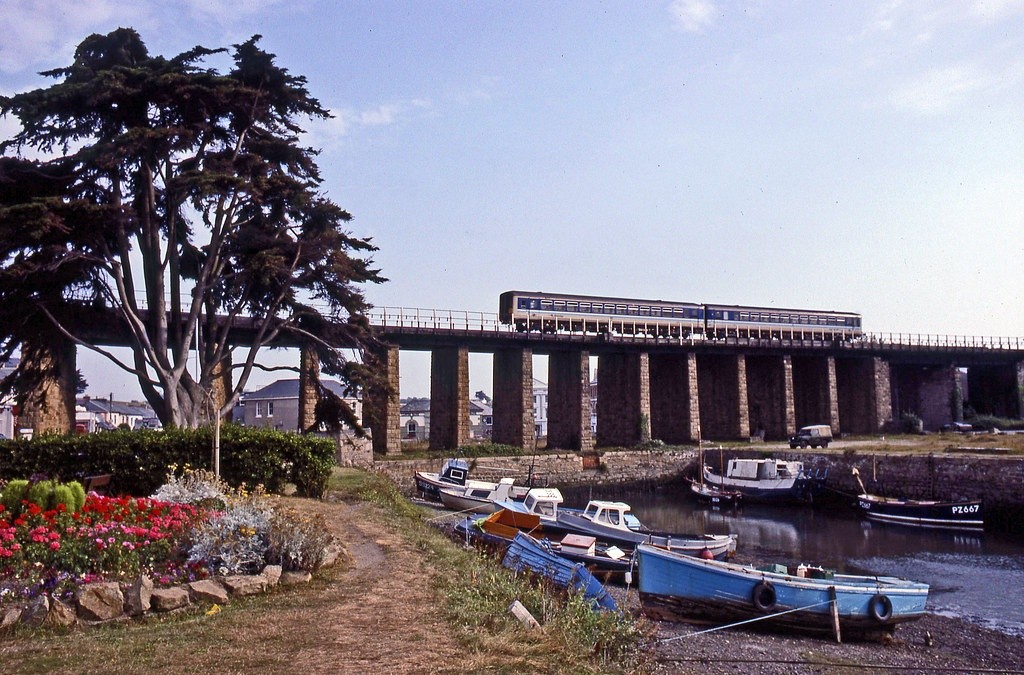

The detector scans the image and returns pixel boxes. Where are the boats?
[684,474,748,508]
[580,499,648,533]
[412,456,565,518]
[857,491,988,533]
[503,530,627,623]
[555,510,740,563]
[702,457,830,502]
[454,509,611,555]
[632,542,930,635]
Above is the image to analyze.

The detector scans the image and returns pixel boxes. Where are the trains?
[499,290,863,339]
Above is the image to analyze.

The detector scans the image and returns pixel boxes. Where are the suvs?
[788,426,838,451]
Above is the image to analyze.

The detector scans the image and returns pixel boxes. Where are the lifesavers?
[868,594,893,623]
[752,580,777,612]
